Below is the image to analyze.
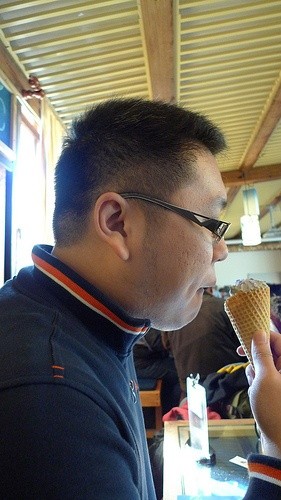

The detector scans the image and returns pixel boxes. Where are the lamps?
[240,177,261,247]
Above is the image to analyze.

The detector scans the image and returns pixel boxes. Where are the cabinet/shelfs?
[163,417,262,500]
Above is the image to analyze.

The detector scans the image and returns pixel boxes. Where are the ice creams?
[224,277,270,373]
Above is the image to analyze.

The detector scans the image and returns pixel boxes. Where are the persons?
[0,92,281,500]
[161,286,247,407]
[133,326,180,429]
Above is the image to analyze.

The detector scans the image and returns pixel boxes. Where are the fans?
[225,206,281,244]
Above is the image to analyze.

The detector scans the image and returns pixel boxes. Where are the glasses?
[120,192,232,250]
